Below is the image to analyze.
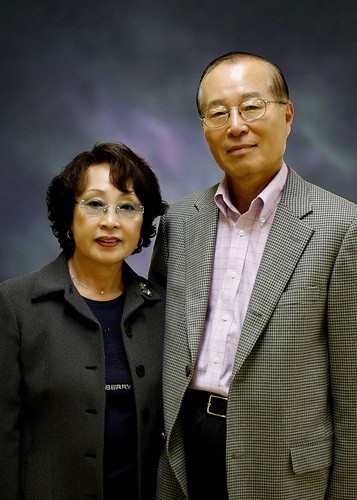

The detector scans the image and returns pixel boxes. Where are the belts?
[183,388,228,418]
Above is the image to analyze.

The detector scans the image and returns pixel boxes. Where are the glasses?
[198,98,286,128]
[76,199,144,221]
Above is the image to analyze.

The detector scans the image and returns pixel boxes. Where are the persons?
[0,141,170,500]
[147,51,357,500]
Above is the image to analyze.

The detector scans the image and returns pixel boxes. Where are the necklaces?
[70,272,121,294]
